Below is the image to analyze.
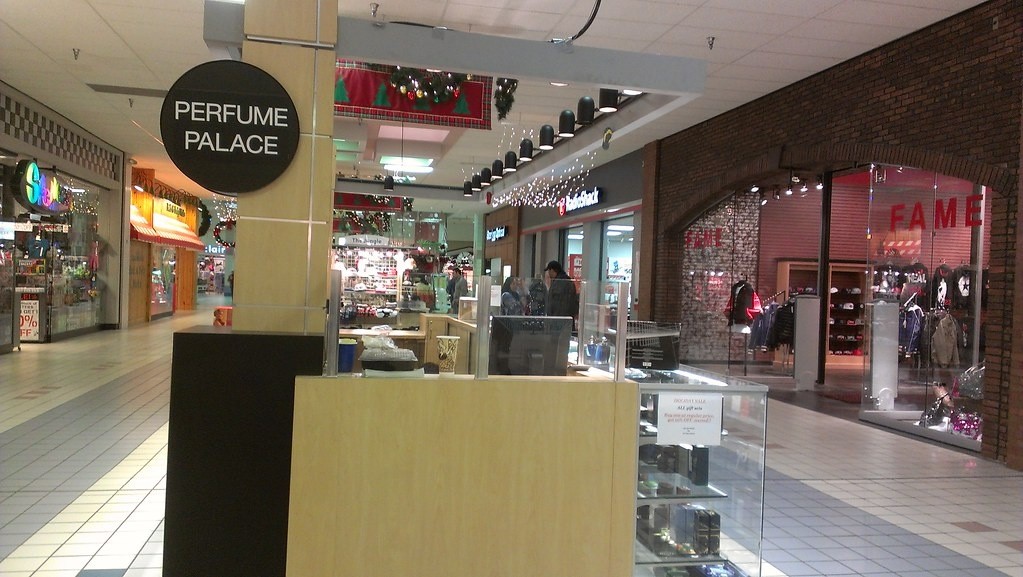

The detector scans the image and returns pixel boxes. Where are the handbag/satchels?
[919,397,943,427]
[957,365,984,398]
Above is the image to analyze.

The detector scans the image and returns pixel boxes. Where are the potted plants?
[412,239,450,273]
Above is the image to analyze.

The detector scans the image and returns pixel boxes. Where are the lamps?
[750,184,759,193]
[800,183,809,192]
[464,181,473,197]
[384,175,394,192]
[472,175,482,192]
[505,151,520,172]
[539,125,559,151]
[520,139,541,161]
[758,189,768,206]
[815,179,823,191]
[491,160,505,179]
[558,109,580,138]
[773,187,781,200]
[577,96,599,126]
[481,167,491,186]
[785,184,793,195]
[598,88,625,112]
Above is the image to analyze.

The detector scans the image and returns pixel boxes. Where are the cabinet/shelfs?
[591,364,769,577]
[420,313,478,374]
[774,256,877,369]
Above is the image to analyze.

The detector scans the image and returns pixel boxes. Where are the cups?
[339,343,357,374]
[436,335,460,374]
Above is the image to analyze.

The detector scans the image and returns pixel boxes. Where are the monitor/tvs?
[492,316,572,376]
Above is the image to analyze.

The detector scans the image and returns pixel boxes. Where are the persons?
[227,268,234,297]
[410,268,468,315]
[500,260,578,337]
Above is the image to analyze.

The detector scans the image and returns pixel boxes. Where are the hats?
[544,261,561,271]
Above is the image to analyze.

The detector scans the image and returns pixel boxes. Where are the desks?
[339,329,424,353]
